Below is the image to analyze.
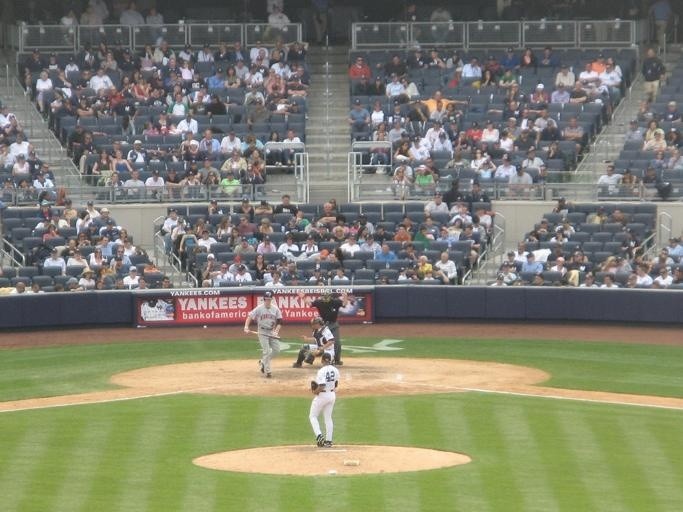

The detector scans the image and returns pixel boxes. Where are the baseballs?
[202,325,207,328]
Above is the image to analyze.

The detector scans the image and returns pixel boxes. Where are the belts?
[321,389,332,392]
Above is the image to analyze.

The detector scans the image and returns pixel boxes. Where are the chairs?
[495,196,658,287]
[151,200,506,289]
[0,92,170,295]
[344,43,683,203]
[15,40,314,198]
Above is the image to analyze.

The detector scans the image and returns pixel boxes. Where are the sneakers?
[317,435,324,447]
[325,441,332,447]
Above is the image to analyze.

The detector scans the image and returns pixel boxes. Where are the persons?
[351,45,622,196]
[0,105,70,207]
[308,352,339,447]
[494,198,682,287]
[243,291,283,378]
[297,289,349,365]
[617,0,673,44]
[496,0,513,42]
[233,0,335,44]
[13,200,171,293]
[24,42,310,198]
[292,316,334,367]
[163,182,496,288]
[598,49,682,197]
[361,2,452,49]
[60,0,167,46]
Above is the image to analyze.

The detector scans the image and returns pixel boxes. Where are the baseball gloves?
[311,381,319,395]
[310,346,323,356]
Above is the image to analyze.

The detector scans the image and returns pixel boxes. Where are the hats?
[322,353,331,361]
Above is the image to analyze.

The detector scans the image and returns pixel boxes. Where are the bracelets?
[274,328,279,332]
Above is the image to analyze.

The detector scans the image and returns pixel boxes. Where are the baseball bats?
[244,329,280,340]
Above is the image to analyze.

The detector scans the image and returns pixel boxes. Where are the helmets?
[264,291,330,323]
